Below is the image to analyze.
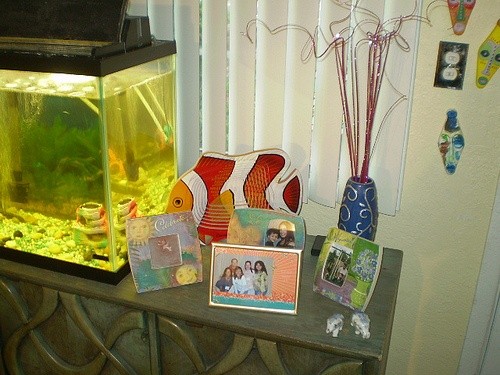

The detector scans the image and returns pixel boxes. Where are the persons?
[264,229,277,248]
[329,261,348,287]
[277,222,296,248]
[216,258,268,296]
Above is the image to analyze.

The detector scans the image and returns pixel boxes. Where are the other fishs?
[164,147,304,245]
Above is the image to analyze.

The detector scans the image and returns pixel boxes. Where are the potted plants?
[246,1,460,238]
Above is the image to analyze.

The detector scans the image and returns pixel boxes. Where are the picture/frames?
[209,241,302,316]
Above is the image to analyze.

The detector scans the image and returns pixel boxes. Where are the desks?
[0,234,404,373]
